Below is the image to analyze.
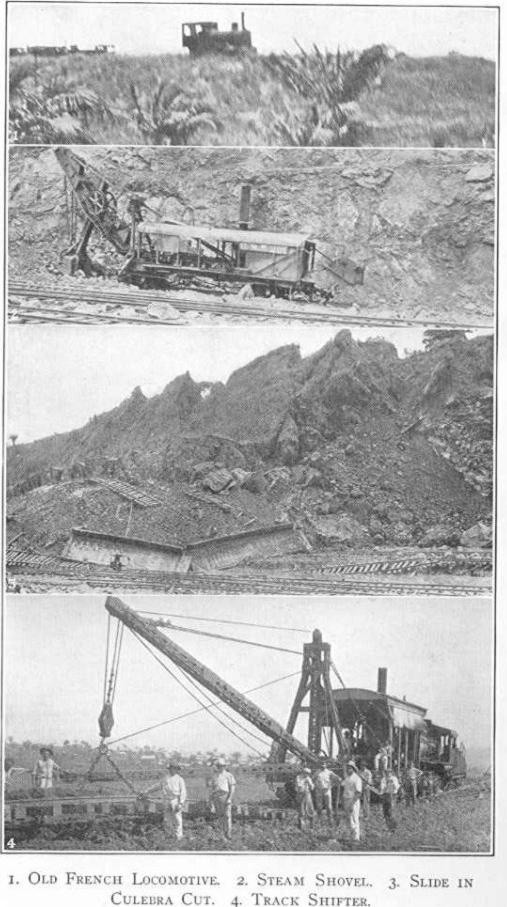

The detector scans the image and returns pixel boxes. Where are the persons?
[33,748,62,788]
[204,758,236,840]
[294,728,423,841]
[4,758,25,779]
[142,761,187,837]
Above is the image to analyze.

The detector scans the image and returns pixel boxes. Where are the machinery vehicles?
[46,145,365,309]
[83,595,474,822]
[173,10,259,56]
